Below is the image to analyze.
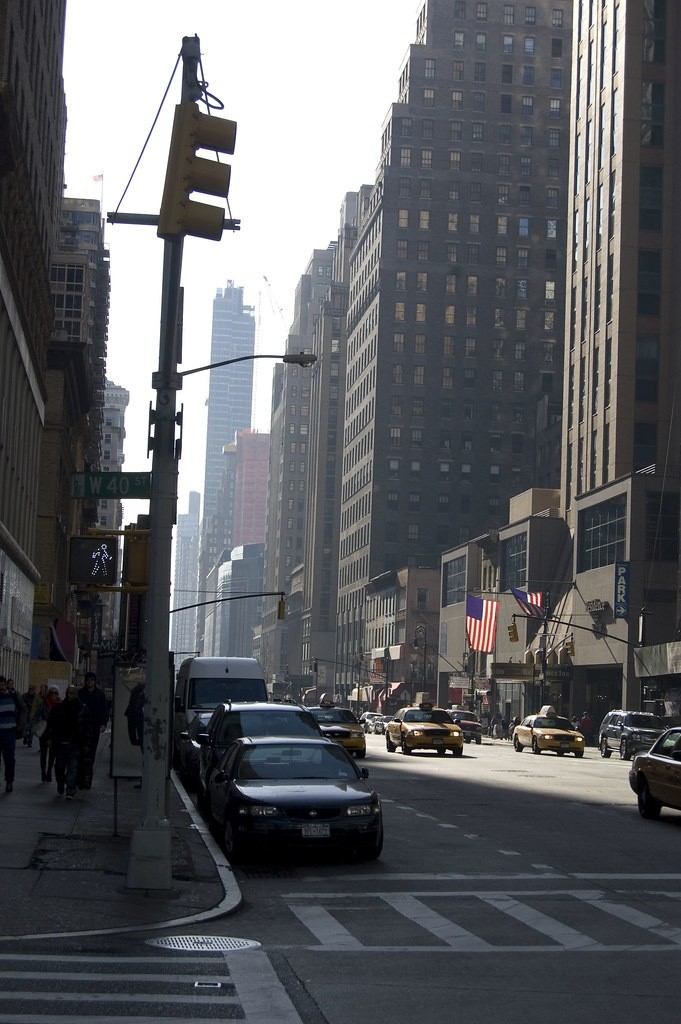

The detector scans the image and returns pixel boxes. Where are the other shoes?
[22,734,32,747]
[42,776,52,784]
[56,791,74,800]
[5,781,12,792]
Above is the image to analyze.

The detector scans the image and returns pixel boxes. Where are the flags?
[505,585,547,618]
[465,593,498,654]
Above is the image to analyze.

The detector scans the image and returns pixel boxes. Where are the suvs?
[195,698,323,823]
[598,709,670,760]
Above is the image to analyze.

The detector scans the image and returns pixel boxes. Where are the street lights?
[412,626,427,692]
[145,350,317,831]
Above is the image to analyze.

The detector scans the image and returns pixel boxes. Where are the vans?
[173,656,282,793]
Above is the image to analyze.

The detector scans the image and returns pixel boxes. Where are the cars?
[629,726,681,820]
[358,711,394,735]
[387,692,464,758]
[205,736,384,869]
[445,710,482,744]
[304,692,366,759]
[512,704,585,758]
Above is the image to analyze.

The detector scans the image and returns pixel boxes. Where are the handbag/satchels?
[30,720,47,737]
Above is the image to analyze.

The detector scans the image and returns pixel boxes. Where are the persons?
[571,716,582,730]
[581,712,593,747]
[0,675,21,792]
[30,686,63,782]
[121,681,147,767]
[49,685,89,799]
[78,671,108,789]
[6,679,48,747]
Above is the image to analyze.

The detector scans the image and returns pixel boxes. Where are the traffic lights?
[565,641,575,657]
[69,535,118,585]
[508,623,519,643]
[156,100,238,243]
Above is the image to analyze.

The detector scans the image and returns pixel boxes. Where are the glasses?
[50,692,58,695]
[29,688,35,691]
